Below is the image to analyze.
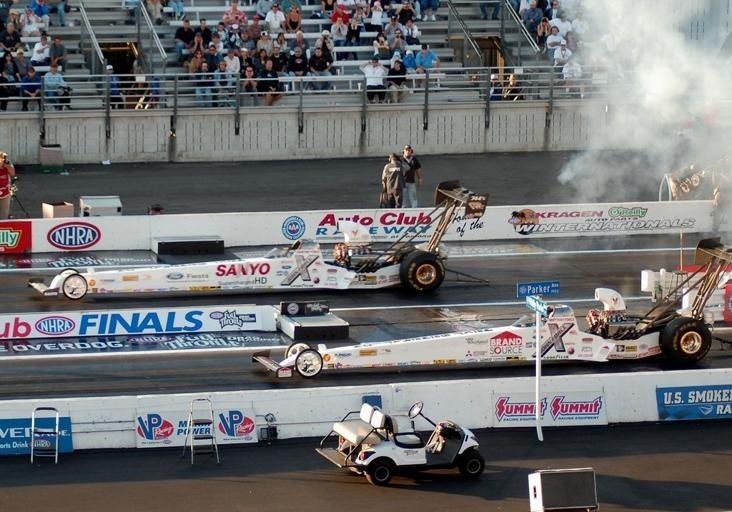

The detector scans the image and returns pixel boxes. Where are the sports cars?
[25,179,495,299]
[246,231,732,376]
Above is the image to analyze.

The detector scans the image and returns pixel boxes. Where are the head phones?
[402,145,414,154]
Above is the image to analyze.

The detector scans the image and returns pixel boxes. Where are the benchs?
[332,401,386,444]
[0,1,613,110]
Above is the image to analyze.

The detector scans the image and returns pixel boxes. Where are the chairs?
[30,407,60,466]
[181,398,221,465]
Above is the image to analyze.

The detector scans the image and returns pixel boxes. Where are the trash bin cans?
[41,201,75,217]
[39,144,66,173]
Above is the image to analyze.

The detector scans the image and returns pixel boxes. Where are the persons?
[1,152,15,219]
[479,0,630,100]
[330,243,346,267]
[584,309,605,338]
[382,152,411,209]
[399,145,423,209]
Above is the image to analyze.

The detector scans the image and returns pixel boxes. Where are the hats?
[16,47,24,55]
[391,15,396,20]
[106,65,114,70]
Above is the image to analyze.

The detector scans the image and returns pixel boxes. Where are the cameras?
[4,154,9,164]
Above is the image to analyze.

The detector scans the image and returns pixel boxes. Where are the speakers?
[527,467,599,512]
